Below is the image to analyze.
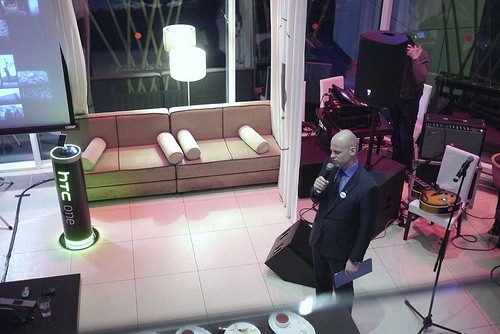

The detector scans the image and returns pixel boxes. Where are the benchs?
[169,99,280,192]
[58,108,177,201]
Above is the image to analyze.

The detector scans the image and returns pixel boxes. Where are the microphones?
[316,162,334,195]
[452,156,474,182]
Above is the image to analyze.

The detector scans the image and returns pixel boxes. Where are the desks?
[316,108,393,154]
[0,272,81,334]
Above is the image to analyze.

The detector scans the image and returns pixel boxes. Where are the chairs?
[413,84,433,141]
[403,145,480,253]
[320,76,344,131]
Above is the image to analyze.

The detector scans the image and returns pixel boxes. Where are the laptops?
[0,298,37,323]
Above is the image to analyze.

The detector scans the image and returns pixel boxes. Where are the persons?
[391,33,429,183]
[4,61,11,84]
[309,129,380,316]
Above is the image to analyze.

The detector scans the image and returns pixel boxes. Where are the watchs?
[349,259,362,265]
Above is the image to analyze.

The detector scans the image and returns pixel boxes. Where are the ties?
[327,169,343,208]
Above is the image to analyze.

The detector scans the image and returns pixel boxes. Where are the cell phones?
[57,134,67,146]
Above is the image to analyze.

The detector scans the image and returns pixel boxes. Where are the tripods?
[405,174,466,334]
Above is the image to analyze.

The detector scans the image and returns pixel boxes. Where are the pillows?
[176,128,201,160]
[157,131,185,165]
[80,137,106,170]
[235,124,270,154]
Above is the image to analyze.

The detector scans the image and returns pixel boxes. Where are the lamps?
[169,47,207,106]
[163,24,197,51]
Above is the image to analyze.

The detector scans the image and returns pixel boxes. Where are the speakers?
[354,29,411,109]
[417,113,487,162]
[265,219,316,288]
[355,149,407,239]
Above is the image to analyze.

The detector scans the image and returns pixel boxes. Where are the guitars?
[405,170,462,214]
